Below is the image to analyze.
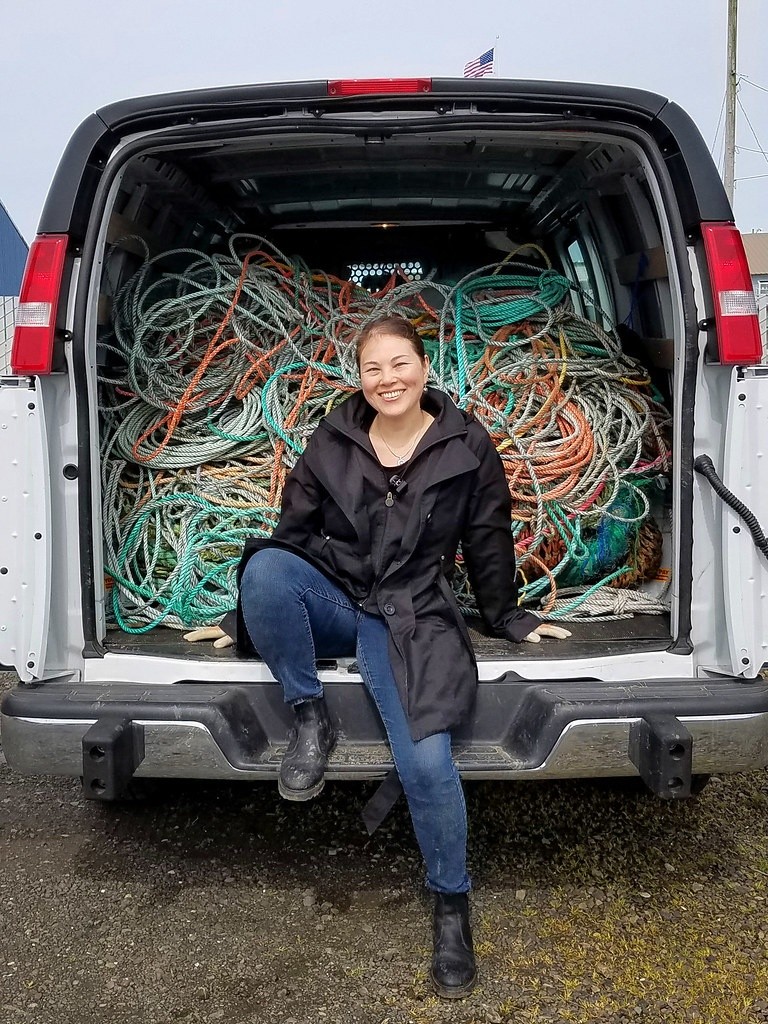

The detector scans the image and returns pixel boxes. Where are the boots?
[430,888,478,999]
[276,692,334,799]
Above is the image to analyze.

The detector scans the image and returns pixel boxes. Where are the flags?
[463,47,494,78]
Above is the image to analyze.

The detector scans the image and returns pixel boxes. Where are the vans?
[0,58,766,811]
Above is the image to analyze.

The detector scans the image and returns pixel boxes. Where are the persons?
[184,316,572,997]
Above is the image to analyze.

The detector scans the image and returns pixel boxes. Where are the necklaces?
[377,408,424,465]
